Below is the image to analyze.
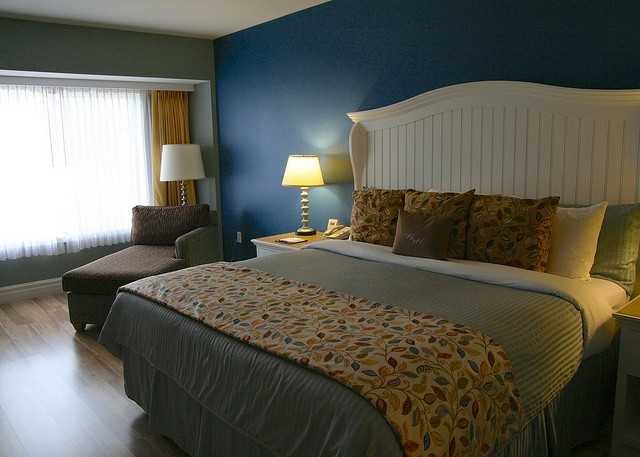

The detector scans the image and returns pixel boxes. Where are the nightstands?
[610,295,640,456]
[250,229,331,258]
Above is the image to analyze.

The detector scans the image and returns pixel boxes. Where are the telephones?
[323,225,351,240]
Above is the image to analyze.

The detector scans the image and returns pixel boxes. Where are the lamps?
[281,154,324,235]
[159,143,207,205]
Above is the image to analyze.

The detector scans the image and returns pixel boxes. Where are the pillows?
[405,189,475,260]
[349,189,406,247]
[546,201,608,279]
[392,209,452,260]
[466,194,561,272]
[560,202,640,296]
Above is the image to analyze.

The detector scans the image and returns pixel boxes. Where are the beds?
[97,80,638,456]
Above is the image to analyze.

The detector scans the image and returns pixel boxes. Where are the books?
[275,238,307,244]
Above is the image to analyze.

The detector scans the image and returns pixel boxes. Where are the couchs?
[61,204,219,330]
